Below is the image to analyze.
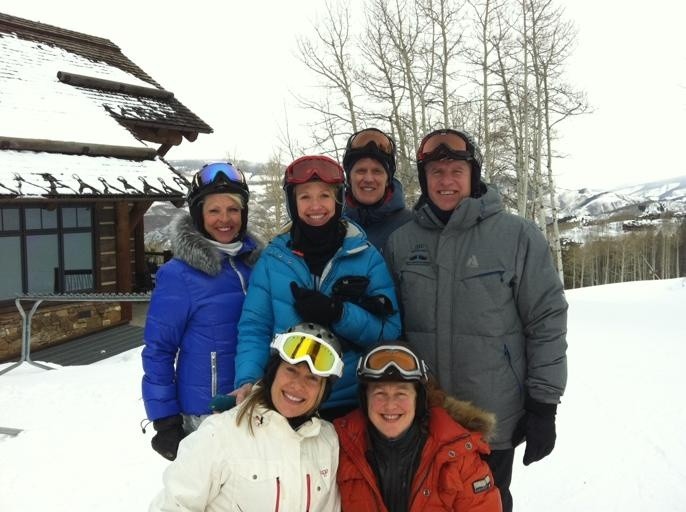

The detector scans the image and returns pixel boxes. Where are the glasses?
[419,131,471,155]
[363,349,421,373]
[349,128,393,154]
[194,162,244,182]
[269,334,343,377]
[287,155,343,183]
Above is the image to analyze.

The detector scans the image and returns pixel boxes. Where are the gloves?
[512,401,556,465]
[335,275,391,310]
[292,283,341,323]
[151,418,186,460]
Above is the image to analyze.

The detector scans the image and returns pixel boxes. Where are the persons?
[334,341,503,512]
[227,154,405,421]
[343,127,413,257]
[384,126,570,512]
[159,322,343,509]
[139,161,266,460]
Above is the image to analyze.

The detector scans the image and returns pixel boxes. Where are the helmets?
[263,324,343,404]
[343,130,395,187]
[417,129,484,206]
[189,166,249,233]
[356,344,428,419]
[283,159,346,220]
